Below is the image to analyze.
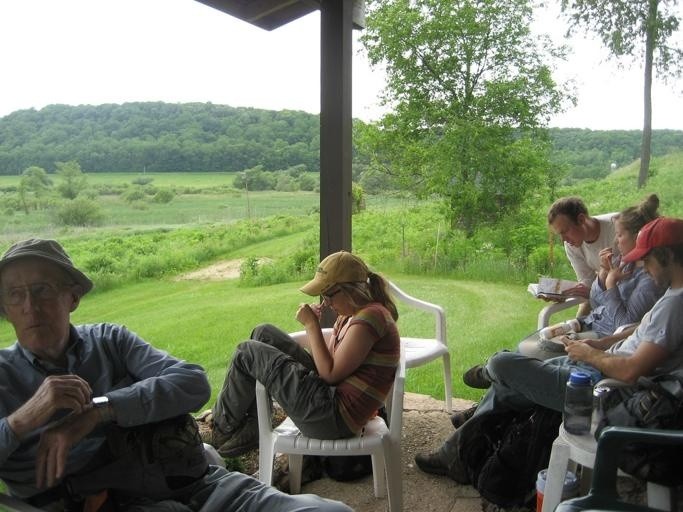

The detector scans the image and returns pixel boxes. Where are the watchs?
[92,396,114,426]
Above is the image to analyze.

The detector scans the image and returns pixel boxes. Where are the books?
[526,277,580,303]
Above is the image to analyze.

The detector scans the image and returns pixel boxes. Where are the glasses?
[320,287,341,301]
[2,281,75,306]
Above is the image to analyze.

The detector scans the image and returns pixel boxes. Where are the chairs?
[378,273,451,415]
[537,295,682,512]
[255,329,406,512]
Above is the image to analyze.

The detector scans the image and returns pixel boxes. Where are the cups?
[563,370,594,436]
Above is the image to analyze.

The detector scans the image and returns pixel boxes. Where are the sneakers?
[451,406,478,429]
[464,367,491,388]
[415,449,474,485]
[200,420,259,459]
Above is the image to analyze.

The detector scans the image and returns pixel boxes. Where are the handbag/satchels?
[595,370,682,488]
[111,414,206,499]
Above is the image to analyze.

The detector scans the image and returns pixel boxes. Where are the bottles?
[539,319,587,341]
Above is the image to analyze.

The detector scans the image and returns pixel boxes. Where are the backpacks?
[478,413,557,510]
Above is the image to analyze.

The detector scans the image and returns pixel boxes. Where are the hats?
[621,218,683,262]
[2,238,93,297]
[299,249,369,295]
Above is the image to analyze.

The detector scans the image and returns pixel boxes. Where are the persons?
[549,198,619,317]
[414,215,683,485]
[198,249,400,460]
[0,240,356,512]
[449,190,665,431]
